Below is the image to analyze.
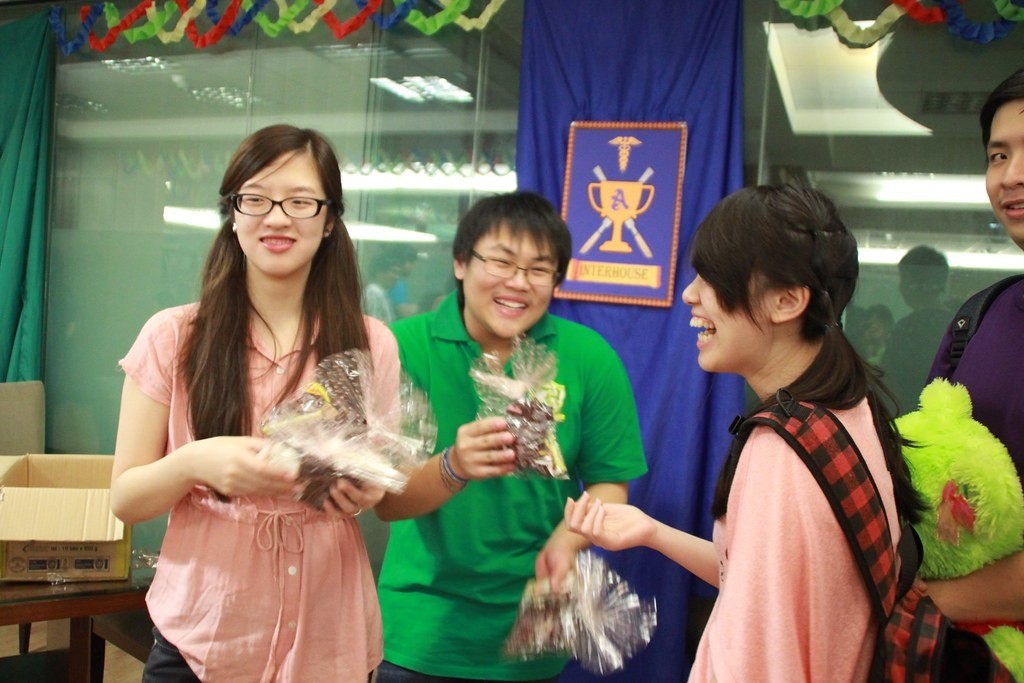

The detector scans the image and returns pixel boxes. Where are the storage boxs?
[0,454,132,585]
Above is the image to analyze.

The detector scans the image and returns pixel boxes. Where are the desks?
[0,581,154,683]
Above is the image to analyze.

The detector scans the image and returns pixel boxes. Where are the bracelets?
[439,446,468,495]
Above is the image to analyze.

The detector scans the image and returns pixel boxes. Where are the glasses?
[228,192,332,220]
[472,247,562,289]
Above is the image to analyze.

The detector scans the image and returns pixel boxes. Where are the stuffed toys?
[890,377,1024,683]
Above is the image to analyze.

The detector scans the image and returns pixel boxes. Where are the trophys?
[589,181,656,252]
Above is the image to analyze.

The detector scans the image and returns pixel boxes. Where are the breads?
[503,576,574,654]
[292,455,363,512]
[500,400,561,476]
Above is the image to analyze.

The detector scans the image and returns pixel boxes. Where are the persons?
[111,122,401,683]
[371,192,649,683]
[564,182,932,683]
[892,66,1024,683]
[845,245,956,419]
[362,242,419,323]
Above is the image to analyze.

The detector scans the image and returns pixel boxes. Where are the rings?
[354,509,362,516]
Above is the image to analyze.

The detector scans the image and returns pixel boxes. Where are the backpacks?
[728,397,1018,683]
